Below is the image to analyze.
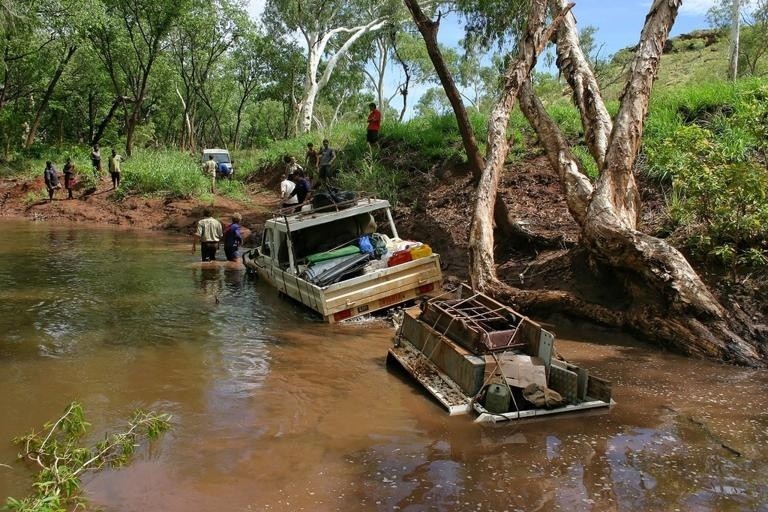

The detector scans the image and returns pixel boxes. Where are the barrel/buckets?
[386,250,412,268]
[358,236,375,256]
[484,382,510,414]
[371,232,387,257]
[407,244,433,261]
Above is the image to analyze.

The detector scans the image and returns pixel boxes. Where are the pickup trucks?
[239,195,443,326]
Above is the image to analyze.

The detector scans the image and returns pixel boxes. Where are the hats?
[203,207,215,217]
[232,213,242,222]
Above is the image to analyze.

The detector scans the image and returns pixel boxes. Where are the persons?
[217,161,231,180]
[44,161,62,201]
[279,174,299,208]
[304,142,320,174]
[224,212,244,263]
[63,158,76,199]
[204,154,219,193]
[285,168,312,205]
[317,138,337,179]
[367,102,382,143]
[90,144,104,181]
[190,205,224,262]
[284,155,302,177]
[108,149,125,188]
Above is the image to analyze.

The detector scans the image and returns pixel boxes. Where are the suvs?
[198,149,233,179]
